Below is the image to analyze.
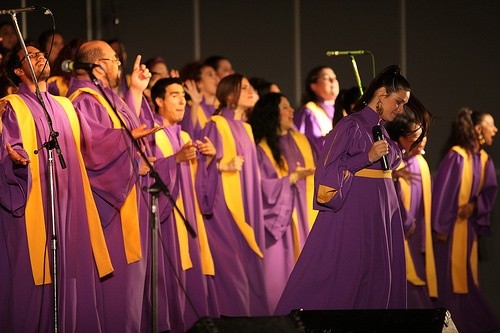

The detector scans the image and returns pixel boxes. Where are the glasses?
[16,52,44,68]
[151,71,169,78]
[98,58,120,64]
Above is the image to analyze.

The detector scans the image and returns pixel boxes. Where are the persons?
[249,78,281,97]
[384,119,438,309]
[332,87,366,128]
[151,77,222,333]
[143,58,179,116]
[396,104,433,135]
[201,55,236,78]
[0,40,114,333]
[272,65,422,315]
[196,74,317,316]
[432,109,498,311]
[248,93,322,316]
[293,65,339,137]
[52,38,88,76]
[39,30,65,76]
[65,42,165,333]
[0,19,19,98]
[178,61,221,140]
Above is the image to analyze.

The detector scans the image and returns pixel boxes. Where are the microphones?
[61,59,98,71]
[0,5,37,14]
[372,125,389,168]
[326,49,370,57]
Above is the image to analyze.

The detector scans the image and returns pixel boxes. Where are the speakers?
[187,307,447,333]
